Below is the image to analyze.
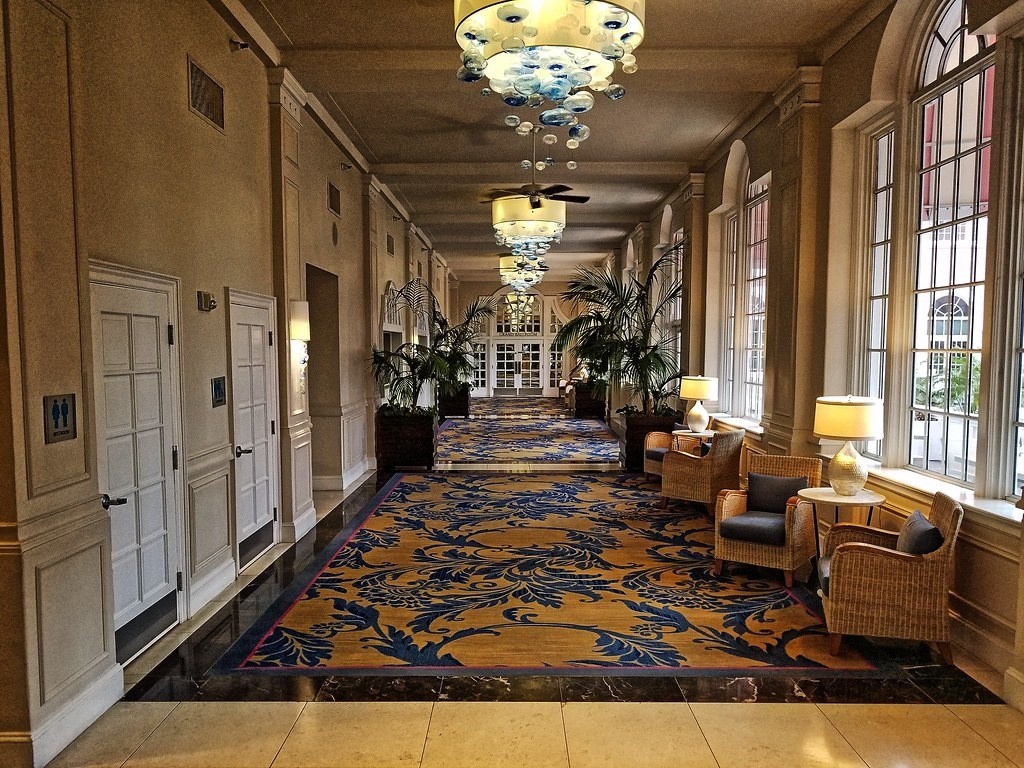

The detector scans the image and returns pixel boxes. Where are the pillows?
[698,441,712,456]
[746,471,808,515]
[895,510,945,554]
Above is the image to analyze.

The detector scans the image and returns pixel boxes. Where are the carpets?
[436,417,621,464]
[203,472,914,680]
[469,396,573,416]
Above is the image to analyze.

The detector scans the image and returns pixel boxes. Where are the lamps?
[812,393,885,496]
[491,195,567,282]
[499,256,546,305]
[679,374,719,433]
[454,0,646,171]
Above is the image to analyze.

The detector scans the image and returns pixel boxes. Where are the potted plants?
[548,236,693,472]
[364,277,499,472]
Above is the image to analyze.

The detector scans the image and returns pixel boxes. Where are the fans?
[478,124,591,210]
[493,256,550,272]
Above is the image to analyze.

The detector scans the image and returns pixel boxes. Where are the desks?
[797,487,887,561]
[671,430,719,451]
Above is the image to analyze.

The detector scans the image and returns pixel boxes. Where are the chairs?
[661,428,746,510]
[713,454,825,588]
[565,384,573,407]
[559,379,568,398]
[815,490,965,667]
[643,413,715,483]
